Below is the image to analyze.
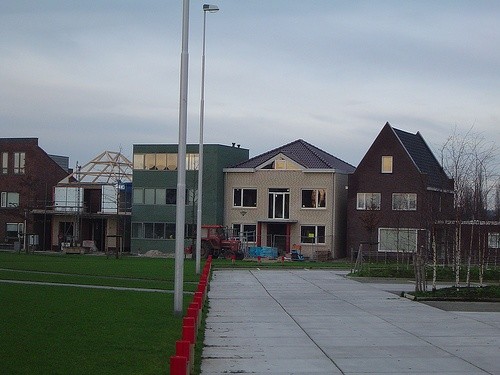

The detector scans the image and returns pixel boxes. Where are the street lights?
[195,3,221,274]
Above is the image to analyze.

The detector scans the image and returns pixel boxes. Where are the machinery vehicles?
[191,225,244,261]
